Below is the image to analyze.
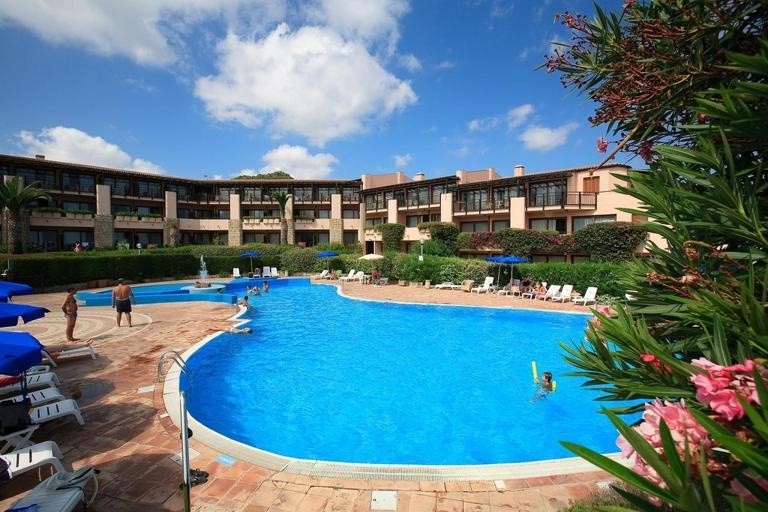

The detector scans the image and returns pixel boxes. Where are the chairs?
[0,343,96,512]
[253,268,260,278]
[262,266,271,277]
[315,270,364,283]
[435,277,597,305]
[271,267,279,277]
[232,268,242,279]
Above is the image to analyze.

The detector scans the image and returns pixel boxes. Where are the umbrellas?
[312,250,340,270]
[0,331,44,376]
[0,302,51,328]
[358,252,384,272]
[240,251,266,272]
[486,255,530,290]
[0,281,32,298]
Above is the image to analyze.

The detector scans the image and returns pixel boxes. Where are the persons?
[61,286,78,340]
[329,268,337,281]
[209,324,252,334]
[519,274,546,300]
[112,278,136,327]
[43,337,94,352]
[75,241,81,252]
[537,372,553,391]
[368,267,381,285]
[194,280,269,307]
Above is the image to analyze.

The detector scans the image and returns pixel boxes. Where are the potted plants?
[417,222,431,233]
[115,211,162,223]
[32,207,93,219]
[249,272,253,278]
[295,216,313,224]
[242,215,280,224]
[242,272,246,277]
[218,270,231,278]
[363,224,382,235]
